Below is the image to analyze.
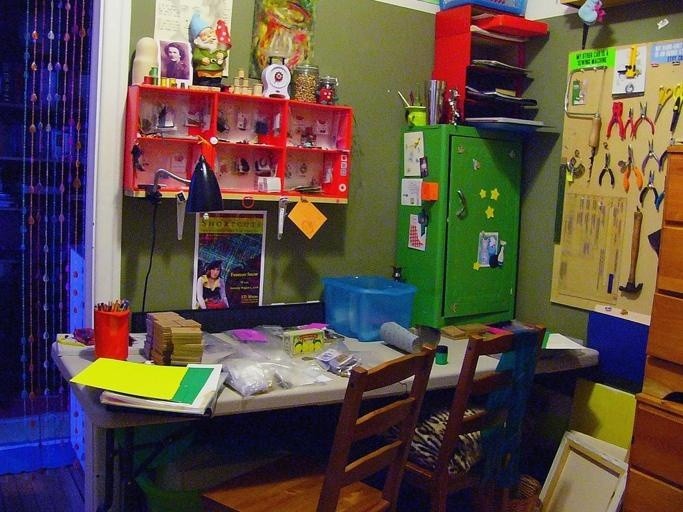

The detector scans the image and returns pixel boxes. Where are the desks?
[48,323,597,511]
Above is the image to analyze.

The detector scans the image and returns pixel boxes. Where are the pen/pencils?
[96,298,130,311]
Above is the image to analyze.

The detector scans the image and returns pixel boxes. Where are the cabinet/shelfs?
[396,123,524,329]
[622,143,682,511]
[124,84,352,207]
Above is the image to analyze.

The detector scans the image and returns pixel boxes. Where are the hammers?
[620,211,646,293]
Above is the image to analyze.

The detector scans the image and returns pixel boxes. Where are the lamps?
[137,155,225,213]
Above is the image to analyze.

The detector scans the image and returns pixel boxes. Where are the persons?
[188,10,234,94]
[196,259,231,311]
[162,41,190,79]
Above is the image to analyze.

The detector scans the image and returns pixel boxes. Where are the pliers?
[639,174,665,212]
[642,138,676,176]
[626,103,655,139]
[606,104,625,140]
[598,152,613,187]
[624,146,642,192]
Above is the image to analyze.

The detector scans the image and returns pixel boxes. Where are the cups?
[425,79,447,125]
[406,106,428,126]
[93,308,130,362]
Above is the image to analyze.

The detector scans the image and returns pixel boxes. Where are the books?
[464,23,546,128]
[93,360,230,417]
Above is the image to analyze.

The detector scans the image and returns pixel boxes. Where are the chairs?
[363,326,547,510]
[199,343,437,509]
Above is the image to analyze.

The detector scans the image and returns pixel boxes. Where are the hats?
[205,260,222,271]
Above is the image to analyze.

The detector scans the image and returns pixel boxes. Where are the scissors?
[653,84,682,138]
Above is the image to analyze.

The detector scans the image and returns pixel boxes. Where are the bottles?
[293,63,338,107]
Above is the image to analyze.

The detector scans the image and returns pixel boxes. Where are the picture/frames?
[539,428,626,511]
